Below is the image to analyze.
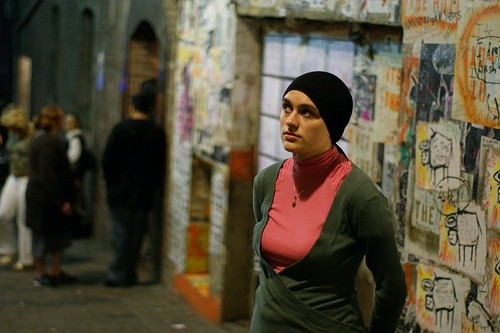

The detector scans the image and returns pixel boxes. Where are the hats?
[283,71,353,144]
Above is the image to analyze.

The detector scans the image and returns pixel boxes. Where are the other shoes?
[34,274,60,285]
[104,277,133,287]
[13,262,33,271]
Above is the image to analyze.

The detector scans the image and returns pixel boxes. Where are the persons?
[62,111,84,172]
[29,105,78,288]
[0,103,34,271]
[101,93,167,288]
[250,71,408,333]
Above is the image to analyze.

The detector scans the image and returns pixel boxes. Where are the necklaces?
[291,160,318,207]
